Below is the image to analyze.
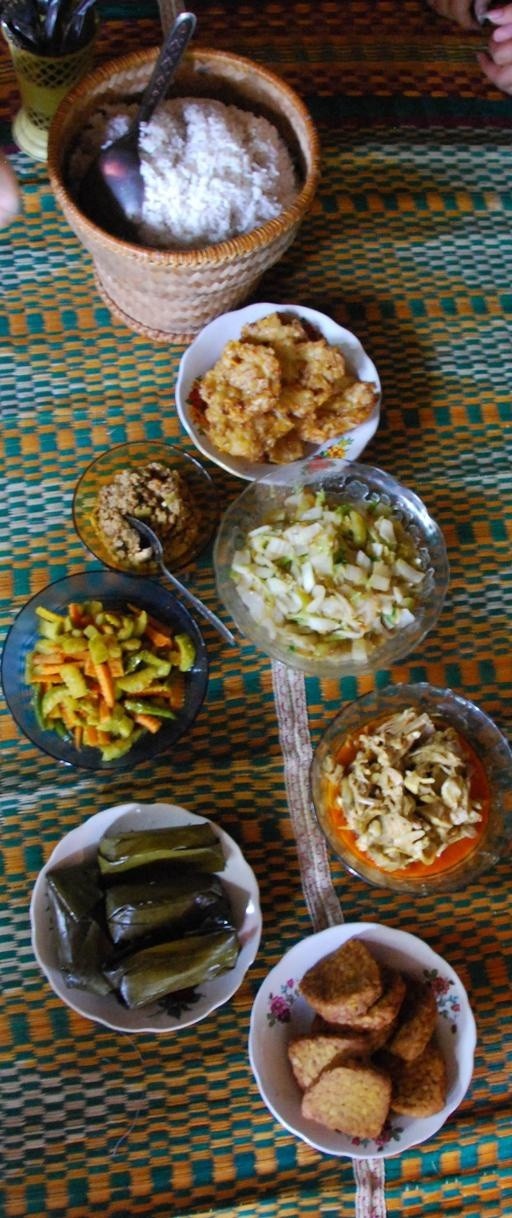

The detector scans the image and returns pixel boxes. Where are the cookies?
[284,938,447,1139]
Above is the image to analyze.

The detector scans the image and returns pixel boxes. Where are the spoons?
[125,513,235,645]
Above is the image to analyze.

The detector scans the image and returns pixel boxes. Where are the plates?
[27,801,266,1035]
[316,684,509,898]
[72,442,216,572]
[177,303,382,488]
[215,460,462,673]
[0,569,212,769]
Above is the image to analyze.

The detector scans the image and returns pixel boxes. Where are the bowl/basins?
[247,919,481,1161]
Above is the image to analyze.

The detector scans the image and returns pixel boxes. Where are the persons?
[0,149,22,229]
[426,0,512,95]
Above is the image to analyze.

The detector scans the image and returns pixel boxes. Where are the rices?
[77,93,300,250]
